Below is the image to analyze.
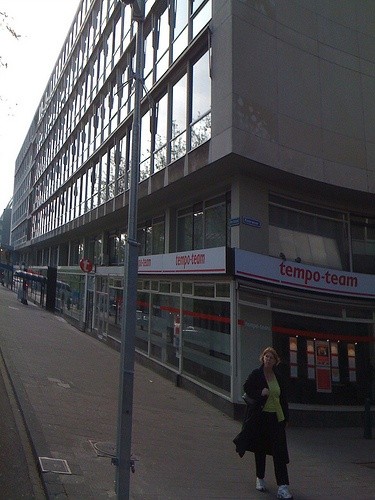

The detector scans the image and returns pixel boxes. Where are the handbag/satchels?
[241,388,264,408]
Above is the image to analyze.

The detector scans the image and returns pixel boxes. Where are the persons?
[242,347,294,499]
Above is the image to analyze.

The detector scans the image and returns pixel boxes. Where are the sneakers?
[255,477,266,492]
[277,485,294,499]
